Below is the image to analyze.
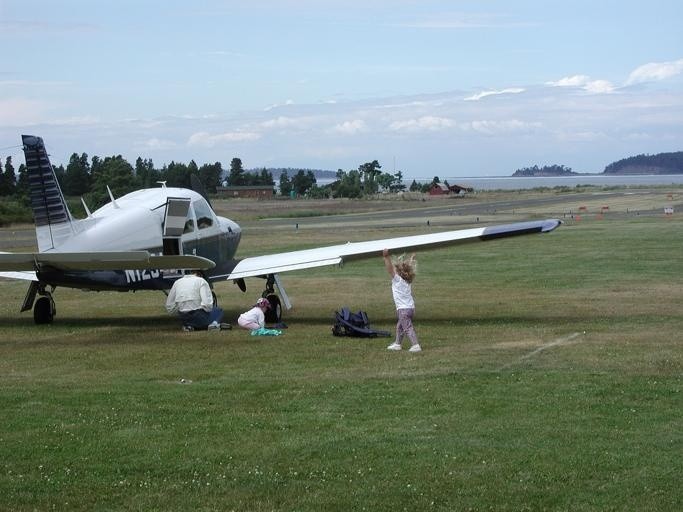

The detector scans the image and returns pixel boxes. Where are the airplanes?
[0,134,563,324]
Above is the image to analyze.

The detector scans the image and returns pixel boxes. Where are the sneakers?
[183,326,194,332]
[209,320,222,330]
[409,343,423,351]
[387,343,402,350]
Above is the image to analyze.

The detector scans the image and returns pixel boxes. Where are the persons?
[164,269,227,331]
[236,297,272,329]
[381,248,424,352]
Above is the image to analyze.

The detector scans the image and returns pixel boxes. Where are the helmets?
[256,298,270,307]
